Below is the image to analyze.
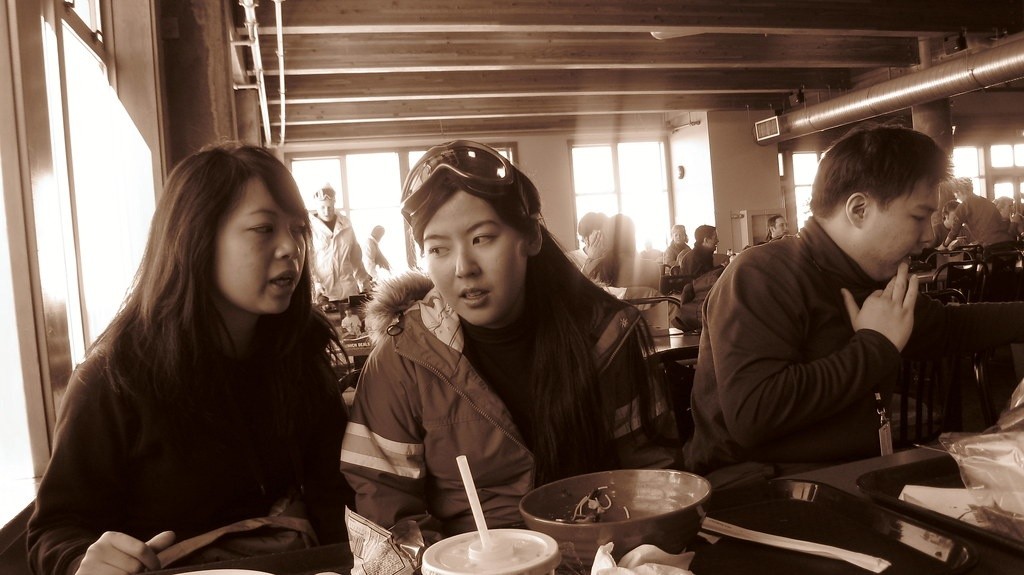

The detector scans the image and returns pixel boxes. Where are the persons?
[301,183,374,314]
[677,124,1024,492]
[338,139,680,549]
[341,305,362,337]
[364,223,391,295]
[581,213,661,297]
[664,174,1024,319]
[562,213,610,274]
[23,141,349,575]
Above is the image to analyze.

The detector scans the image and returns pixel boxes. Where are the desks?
[325,333,376,372]
[131,439,1024,575]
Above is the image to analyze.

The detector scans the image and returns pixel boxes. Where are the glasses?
[312,188,337,201]
[400,139,530,224]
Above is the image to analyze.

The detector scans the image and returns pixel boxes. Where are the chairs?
[889,239,1024,452]
[642,345,702,447]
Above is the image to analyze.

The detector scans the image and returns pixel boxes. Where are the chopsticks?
[700,516,892,573]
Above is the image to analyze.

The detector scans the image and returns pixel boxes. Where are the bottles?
[730,254,737,262]
[727,249,733,256]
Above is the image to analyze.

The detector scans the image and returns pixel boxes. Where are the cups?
[421,528,562,575]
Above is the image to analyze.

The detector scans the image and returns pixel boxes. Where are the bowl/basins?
[518,469,712,569]
